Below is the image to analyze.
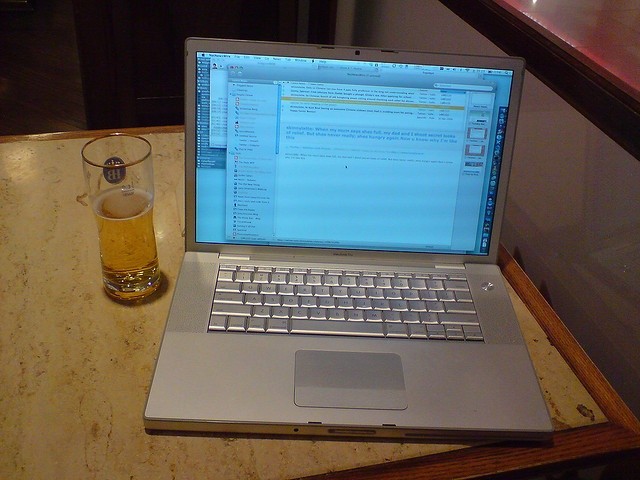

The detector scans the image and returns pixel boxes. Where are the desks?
[1,125,639,480]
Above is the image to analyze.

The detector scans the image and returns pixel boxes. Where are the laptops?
[145,36,555,449]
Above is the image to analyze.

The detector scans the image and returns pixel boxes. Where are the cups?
[80,131,162,309]
